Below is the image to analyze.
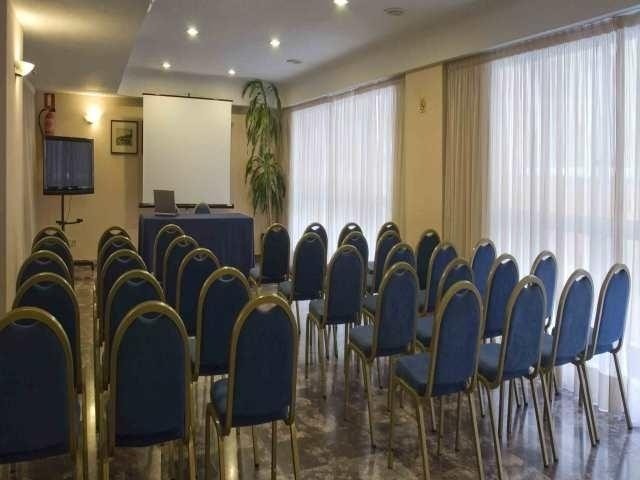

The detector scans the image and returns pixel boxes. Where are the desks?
[138,211,255,269]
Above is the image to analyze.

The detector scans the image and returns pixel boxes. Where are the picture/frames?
[110,120,138,154]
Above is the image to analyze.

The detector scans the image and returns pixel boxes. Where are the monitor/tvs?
[43,135,94,195]
[154,189,176,213]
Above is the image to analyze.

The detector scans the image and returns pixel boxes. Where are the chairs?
[87,224,558,477]
[532,269,599,462]
[194,201,210,213]
[577,262,633,443]
[0,224,84,478]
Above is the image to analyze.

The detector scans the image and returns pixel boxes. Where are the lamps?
[14,60,35,78]
